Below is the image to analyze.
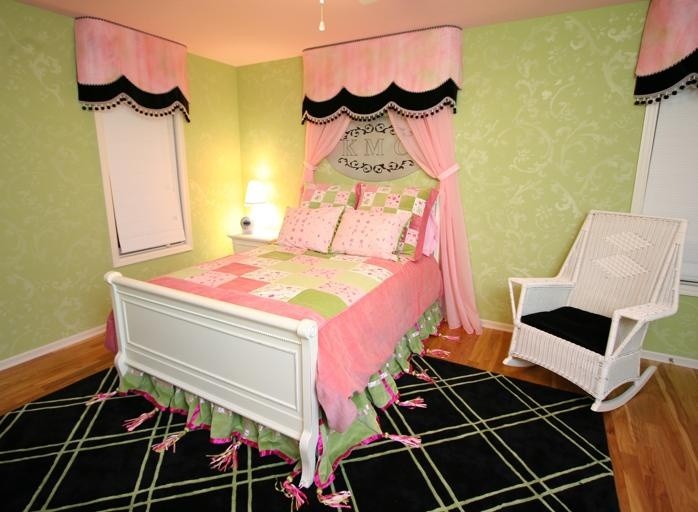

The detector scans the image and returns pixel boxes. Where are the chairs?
[501,208,688,414]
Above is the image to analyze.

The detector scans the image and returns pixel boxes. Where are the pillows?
[275,181,443,263]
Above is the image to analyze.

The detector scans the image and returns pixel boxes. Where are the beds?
[104,242,442,490]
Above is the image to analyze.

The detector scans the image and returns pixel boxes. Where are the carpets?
[1,350,624,511]
